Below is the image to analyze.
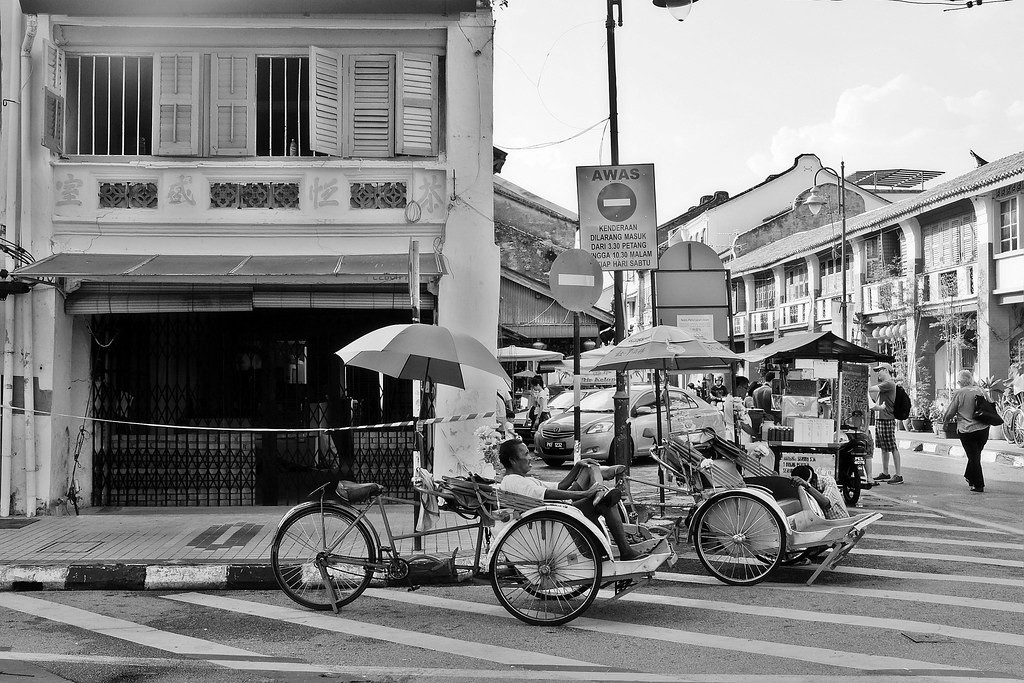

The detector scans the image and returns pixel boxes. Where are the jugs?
[758,421,775,440]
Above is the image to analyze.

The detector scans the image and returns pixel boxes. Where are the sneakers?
[873,473,891,482]
[887,474,903,485]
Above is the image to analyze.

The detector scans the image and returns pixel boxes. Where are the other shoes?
[970,487,983,492]
[965,478,973,487]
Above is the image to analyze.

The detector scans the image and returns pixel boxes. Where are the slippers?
[870,481,879,486]
[622,550,648,560]
[593,488,622,515]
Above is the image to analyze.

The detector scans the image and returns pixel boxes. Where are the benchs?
[704,459,800,521]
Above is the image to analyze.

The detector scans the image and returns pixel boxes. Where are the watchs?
[804,482,812,491]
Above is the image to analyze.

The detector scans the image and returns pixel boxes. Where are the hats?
[873,363,894,373]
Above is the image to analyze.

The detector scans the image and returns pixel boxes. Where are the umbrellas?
[589,319,742,432]
[497,345,565,390]
[333,324,513,420]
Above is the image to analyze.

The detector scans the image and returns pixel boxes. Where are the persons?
[943,370,993,492]
[499,438,650,560]
[515,364,553,462]
[687,371,776,475]
[863,363,904,486]
[789,465,851,550]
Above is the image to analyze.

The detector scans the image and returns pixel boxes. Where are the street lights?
[607,0,697,494]
[802,161,846,342]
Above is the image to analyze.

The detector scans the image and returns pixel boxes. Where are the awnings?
[6,252,449,300]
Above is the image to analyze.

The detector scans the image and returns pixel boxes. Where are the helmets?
[715,376,723,383]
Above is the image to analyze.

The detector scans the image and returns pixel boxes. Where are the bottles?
[768,426,793,441]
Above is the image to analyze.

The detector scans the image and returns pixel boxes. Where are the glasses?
[877,369,884,372]
[956,381,959,384]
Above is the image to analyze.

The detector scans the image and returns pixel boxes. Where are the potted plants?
[863,258,981,437]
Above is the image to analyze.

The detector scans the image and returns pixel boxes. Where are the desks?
[769,441,840,475]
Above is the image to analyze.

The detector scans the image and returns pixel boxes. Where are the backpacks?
[886,385,911,420]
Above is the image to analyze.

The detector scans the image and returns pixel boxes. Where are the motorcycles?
[838,410,872,506]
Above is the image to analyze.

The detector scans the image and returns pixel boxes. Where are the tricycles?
[270,480,677,626]
[595,426,883,595]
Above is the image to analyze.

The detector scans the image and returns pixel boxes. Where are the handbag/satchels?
[972,394,1004,426]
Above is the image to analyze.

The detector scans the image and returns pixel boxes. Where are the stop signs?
[576,164,659,270]
[548,248,603,312]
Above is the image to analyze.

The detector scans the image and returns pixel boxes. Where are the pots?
[746,406,764,433]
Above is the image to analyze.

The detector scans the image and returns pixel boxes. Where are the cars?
[513,389,602,446]
[534,384,725,467]
